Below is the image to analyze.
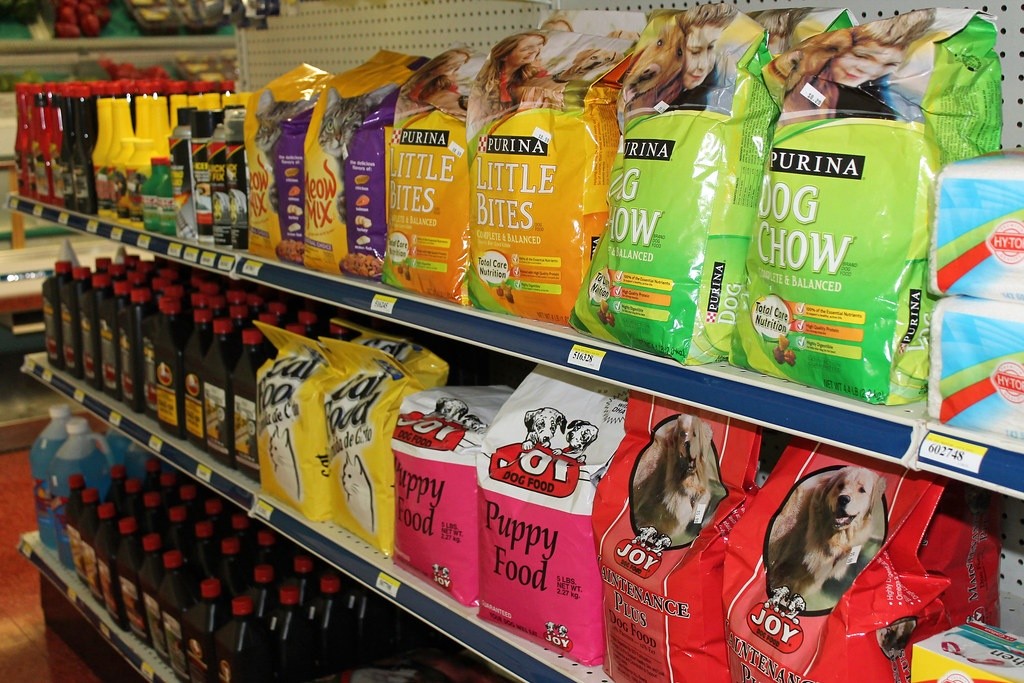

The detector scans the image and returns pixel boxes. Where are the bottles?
[4,76,408,682]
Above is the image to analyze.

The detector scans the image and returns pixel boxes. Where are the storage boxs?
[909,620,1024,683]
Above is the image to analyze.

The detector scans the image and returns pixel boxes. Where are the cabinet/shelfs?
[0,0,1024,683]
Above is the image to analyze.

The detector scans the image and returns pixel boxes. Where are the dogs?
[631,415,714,540]
[766,466,886,611]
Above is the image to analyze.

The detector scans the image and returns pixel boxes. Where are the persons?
[541,18,573,33]
[396,48,469,115]
[664,4,738,111]
[470,32,547,118]
[820,8,936,88]
